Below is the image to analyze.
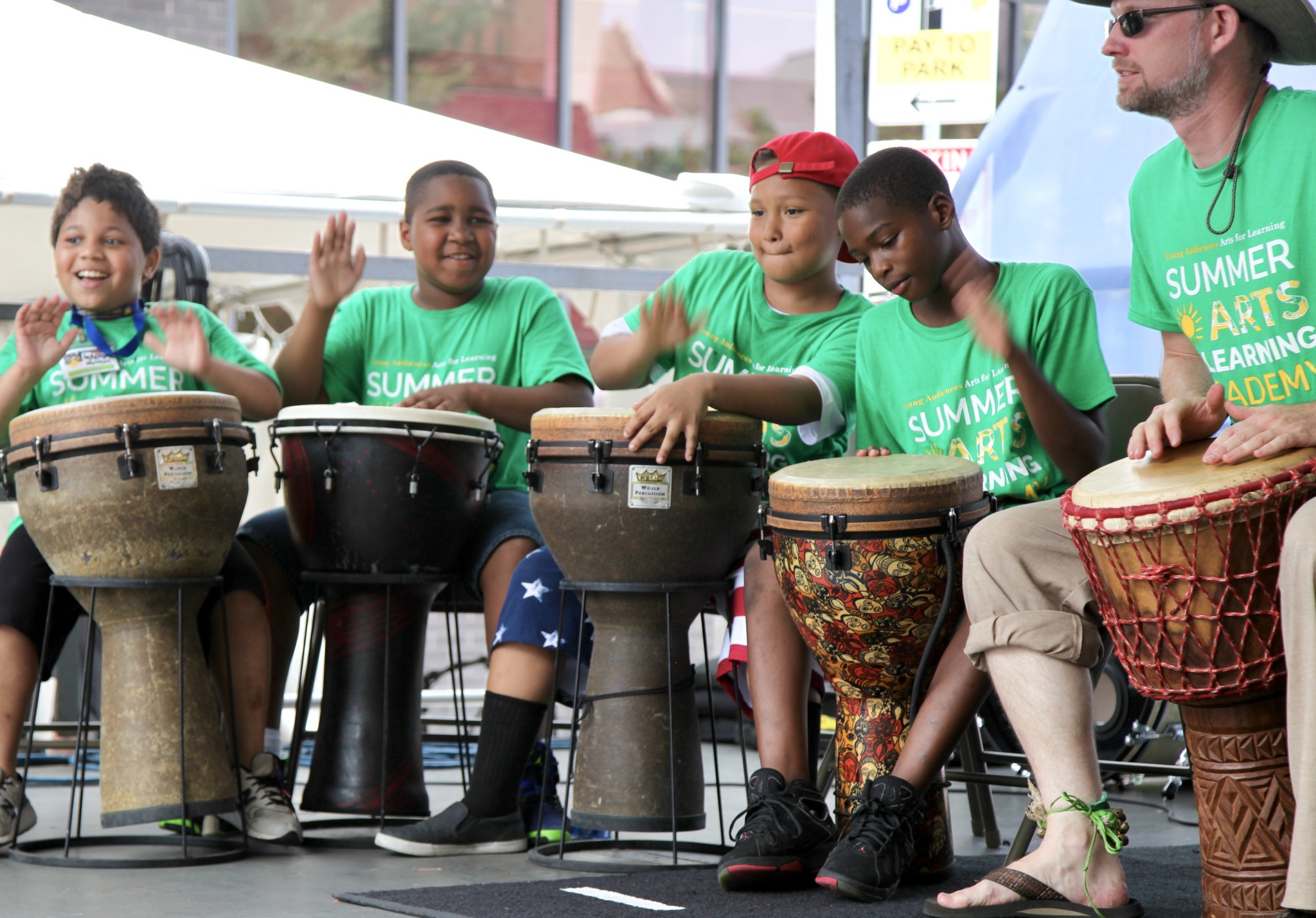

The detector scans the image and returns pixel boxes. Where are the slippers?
[923,867,1143,918]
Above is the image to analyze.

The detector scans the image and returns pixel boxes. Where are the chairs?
[815,377,1193,871]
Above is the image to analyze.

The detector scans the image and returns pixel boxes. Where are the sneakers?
[0,769,37,847]
[518,742,572,846]
[815,775,924,902]
[717,767,838,892]
[214,751,302,843]
[159,818,200,836]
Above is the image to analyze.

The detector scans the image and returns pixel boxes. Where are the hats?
[749,131,861,263]
[1073,1,1315,66]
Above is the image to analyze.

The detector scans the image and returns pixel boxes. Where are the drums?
[524,404,772,832]
[1054,435,1316,918]
[265,396,509,822]
[4,389,254,830]
[766,453,997,871]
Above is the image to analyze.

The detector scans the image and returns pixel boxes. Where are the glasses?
[1107,4,1219,37]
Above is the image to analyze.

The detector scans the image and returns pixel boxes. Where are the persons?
[375,131,875,853]
[924,0,1316,918]
[719,148,1118,902]
[0,164,303,855]
[158,160,594,843]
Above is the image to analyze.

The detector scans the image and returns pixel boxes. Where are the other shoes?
[372,801,528,857]
